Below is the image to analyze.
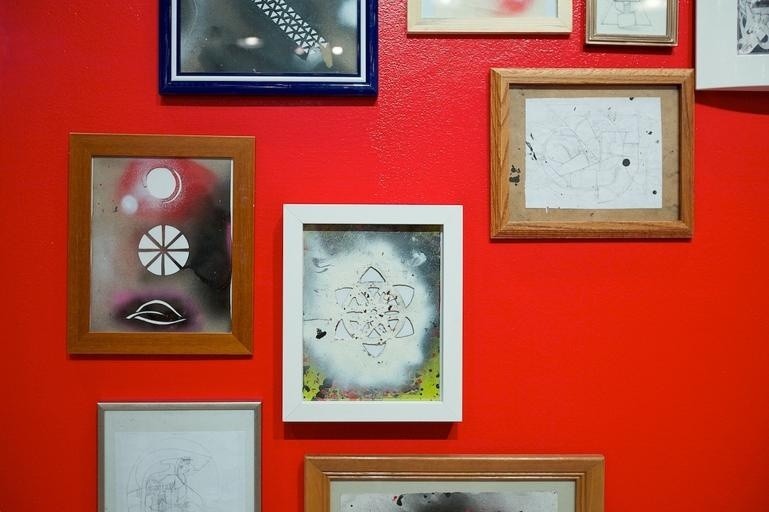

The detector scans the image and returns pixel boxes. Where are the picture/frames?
[66,131,257,358]
[280,203,464,424]
[407,0,574,36]
[691,0,769,96]
[585,0,679,47]
[302,454,606,512]
[157,0,379,98]
[97,399,262,512]
[486,67,695,241]
